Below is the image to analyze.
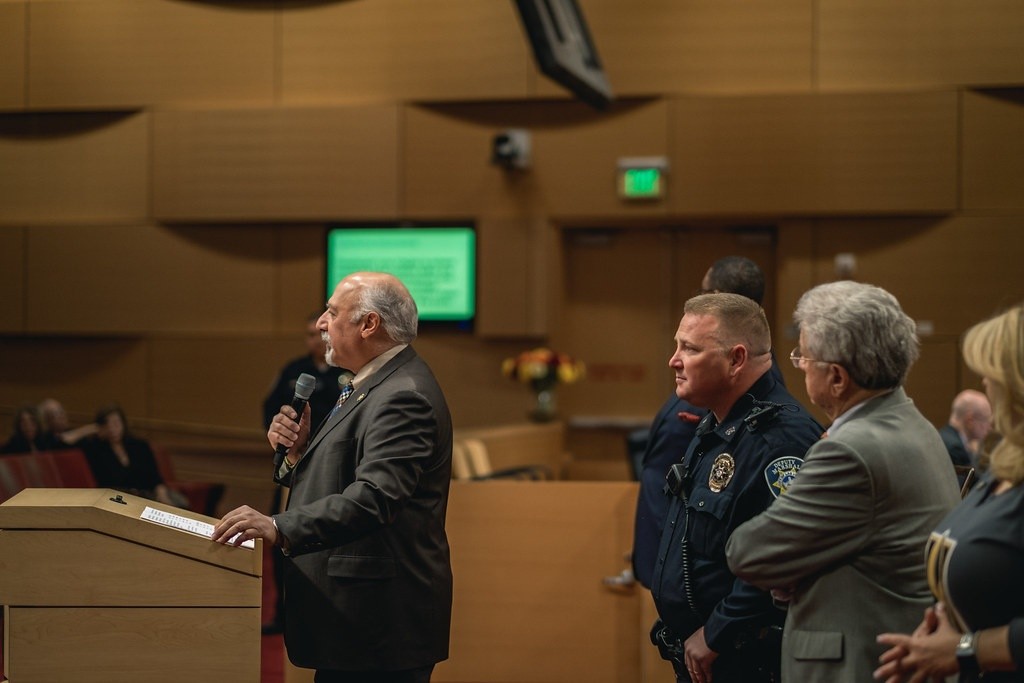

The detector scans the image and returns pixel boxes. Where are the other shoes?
[261,615,284,635]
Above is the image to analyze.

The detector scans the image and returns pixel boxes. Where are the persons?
[632,256,829,683]
[210,271,454,683]
[0,398,178,508]
[261,315,356,636]
[937,389,994,494]
[872,305,1024,683]
[723,279,962,683]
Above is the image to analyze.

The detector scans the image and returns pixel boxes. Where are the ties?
[327,380,354,424]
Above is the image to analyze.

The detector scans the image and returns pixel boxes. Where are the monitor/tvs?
[320,221,480,334]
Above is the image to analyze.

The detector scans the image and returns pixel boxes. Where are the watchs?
[954,628,985,683]
[272,517,283,548]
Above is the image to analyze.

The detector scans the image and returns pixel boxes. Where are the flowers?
[501,347,583,394]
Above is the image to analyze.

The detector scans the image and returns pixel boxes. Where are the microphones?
[273,372,315,470]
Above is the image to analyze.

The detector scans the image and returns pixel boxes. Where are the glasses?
[695,288,715,297]
[790,345,841,368]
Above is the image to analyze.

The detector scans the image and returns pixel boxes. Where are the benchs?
[0,442,227,518]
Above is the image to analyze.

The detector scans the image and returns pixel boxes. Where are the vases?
[523,394,559,424]
